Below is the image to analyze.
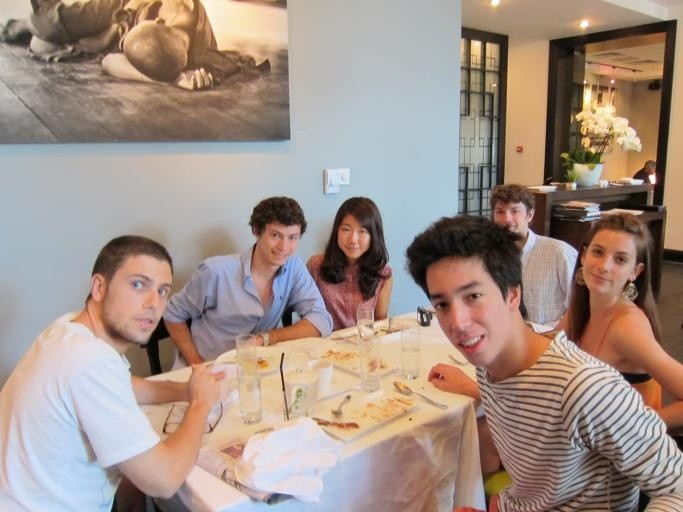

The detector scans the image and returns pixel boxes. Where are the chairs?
[140,316,191,377]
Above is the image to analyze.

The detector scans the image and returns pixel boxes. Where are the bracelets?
[257,331,269,347]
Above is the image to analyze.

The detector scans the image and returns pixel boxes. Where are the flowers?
[559,104,642,187]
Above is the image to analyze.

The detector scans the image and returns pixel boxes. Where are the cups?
[234,333,258,370]
[564,183,576,193]
[280,383,313,420]
[624,180,631,186]
[598,179,607,188]
[307,356,332,392]
[358,334,381,392]
[235,366,261,425]
[355,305,374,340]
[398,324,421,380]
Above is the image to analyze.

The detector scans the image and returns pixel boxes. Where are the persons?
[403,212,682,511]
[534,213,683,446]
[163,196,328,361]
[0,235,219,511]
[302,196,395,333]
[490,183,578,329]
[0,1,227,90]
[624,160,656,205]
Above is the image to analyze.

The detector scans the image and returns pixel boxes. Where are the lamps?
[649,79,660,90]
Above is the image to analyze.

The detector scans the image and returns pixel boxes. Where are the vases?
[567,163,603,187]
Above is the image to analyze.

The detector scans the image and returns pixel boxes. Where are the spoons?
[393,381,451,411]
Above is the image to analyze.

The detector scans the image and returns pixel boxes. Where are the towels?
[204,361,240,420]
[235,415,343,502]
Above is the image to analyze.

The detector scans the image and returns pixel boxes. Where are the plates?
[339,315,414,347]
[313,343,399,380]
[177,390,238,414]
[215,345,287,374]
[309,387,419,442]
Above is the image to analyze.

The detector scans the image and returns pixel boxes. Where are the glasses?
[163,403,223,435]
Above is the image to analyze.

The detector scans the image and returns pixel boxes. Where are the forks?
[447,354,471,367]
[330,394,350,418]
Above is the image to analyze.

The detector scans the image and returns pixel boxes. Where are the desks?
[524,183,667,303]
